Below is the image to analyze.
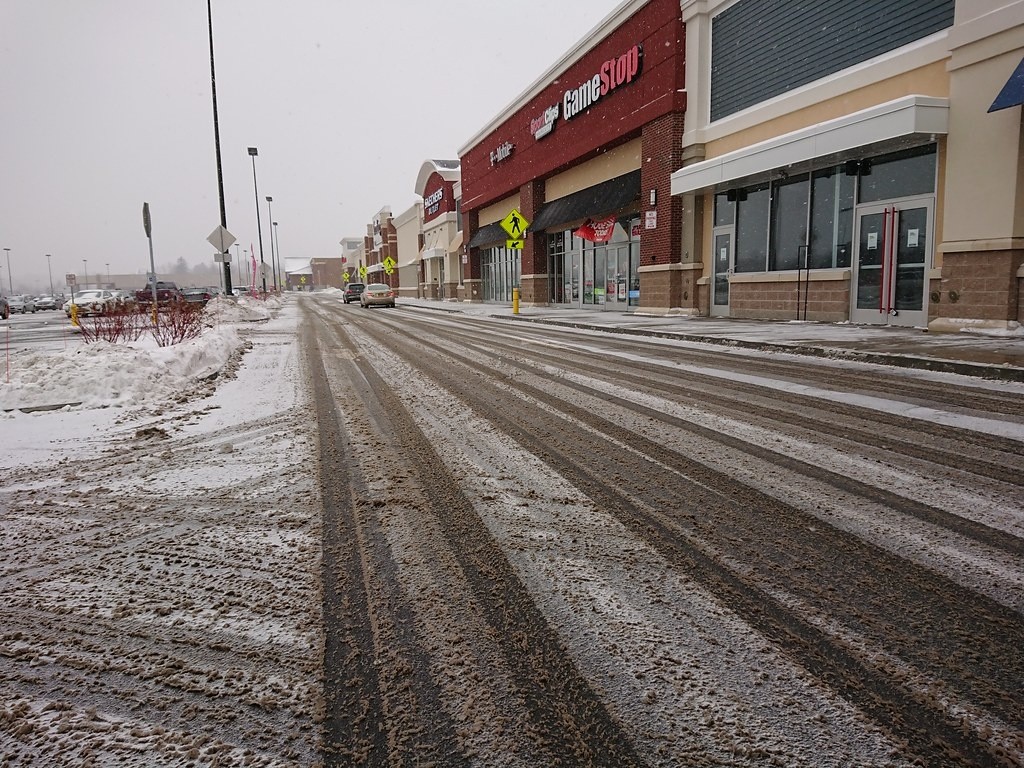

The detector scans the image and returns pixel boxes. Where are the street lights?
[273,221,282,291]
[235,243,242,286]
[243,250,251,286]
[247,145,267,293]
[83,259,89,290]
[265,195,277,290]
[106,263,111,283]
[4,248,13,295]
[45,254,55,296]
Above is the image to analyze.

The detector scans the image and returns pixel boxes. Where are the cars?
[343,283,365,304]
[0,291,75,319]
[359,282,396,308]
[178,287,211,312]
[205,284,259,301]
[62,288,134,319]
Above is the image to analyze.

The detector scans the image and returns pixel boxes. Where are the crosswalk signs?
[359,266,368,275]
[498,208,530,239]
[383,256,396,268]
[343,273,349,279]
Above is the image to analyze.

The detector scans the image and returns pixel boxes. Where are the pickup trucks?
[136,280,178,314]
